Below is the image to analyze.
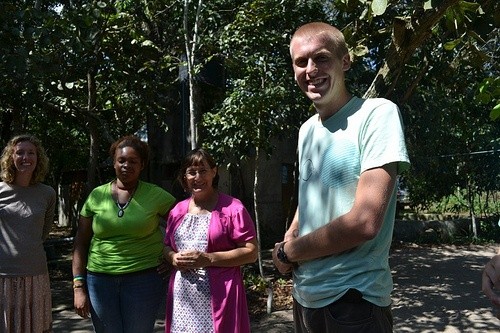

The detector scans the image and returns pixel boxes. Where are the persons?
[70,135,179,333]
[481,253,500,305]
[272,22,412,333]
[1,134,58,333]
[159,146,258,333]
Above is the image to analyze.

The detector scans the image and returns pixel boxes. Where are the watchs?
[277,242,292,264]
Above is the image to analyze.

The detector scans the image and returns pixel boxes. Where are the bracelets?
[73,283,86,289]
[73,273,85,282]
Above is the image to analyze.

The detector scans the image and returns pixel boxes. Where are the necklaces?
[112,177,139,217]
